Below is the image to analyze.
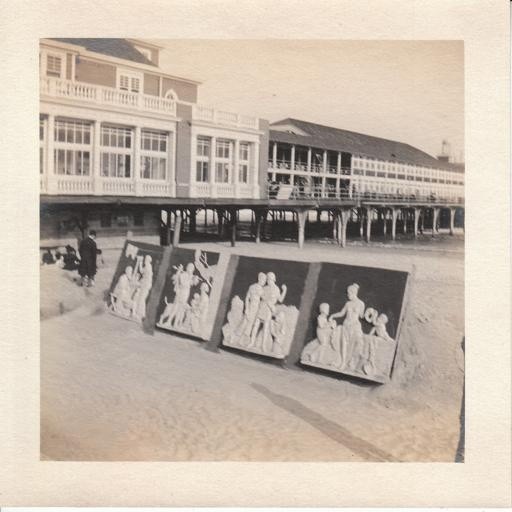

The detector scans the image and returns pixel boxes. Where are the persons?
[269,179,349,201]
[113,254,153,322]
[167,262,209,333]
[233,271,287,352]
[40,244,83,270]
[307,283,396,375]
[78,228,102,288]
